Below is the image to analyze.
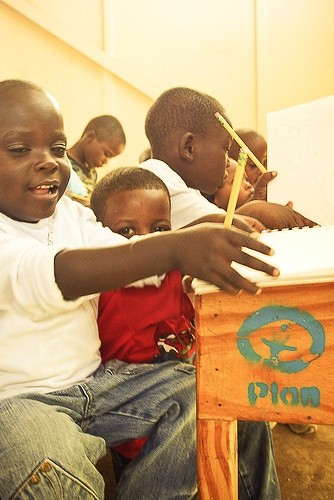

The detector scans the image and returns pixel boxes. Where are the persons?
[66,116,126,197]
[0,79,280,500]
[91,87,321,500]
